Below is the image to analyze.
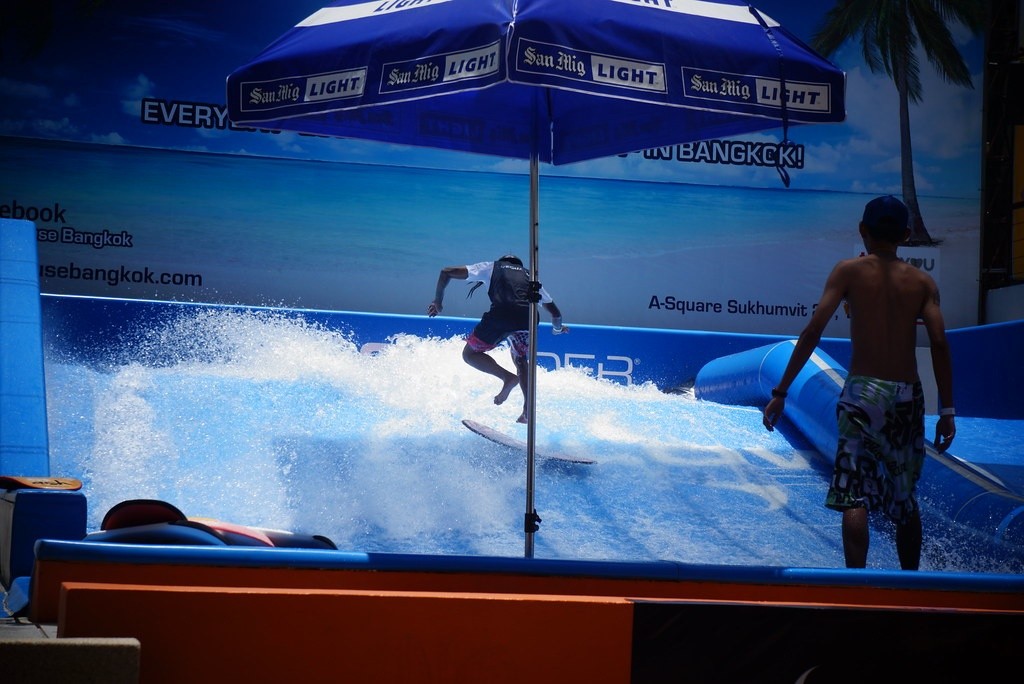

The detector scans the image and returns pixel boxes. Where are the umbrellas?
[226,0,846,559]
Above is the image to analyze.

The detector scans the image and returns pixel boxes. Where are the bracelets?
[553,327,563,333]
[940,408,955,417]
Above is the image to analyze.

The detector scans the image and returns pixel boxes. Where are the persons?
[428,255,569,424]
[763,196,956,570]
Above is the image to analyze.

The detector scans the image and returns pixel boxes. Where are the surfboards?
[462,418,593,465]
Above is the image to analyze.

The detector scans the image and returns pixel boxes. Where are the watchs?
[772,388,788,398]
